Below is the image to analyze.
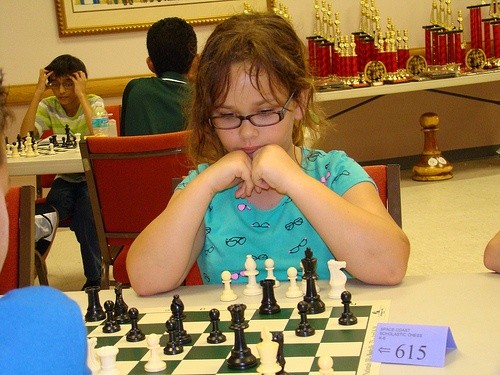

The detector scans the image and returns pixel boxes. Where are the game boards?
[83,296,394,374]
[3,134,81,159]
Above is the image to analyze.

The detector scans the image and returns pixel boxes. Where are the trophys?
[241,0,500,87]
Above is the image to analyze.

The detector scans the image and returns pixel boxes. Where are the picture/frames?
[55,0,275,40]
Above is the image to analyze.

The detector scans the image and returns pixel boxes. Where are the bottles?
[92,102,109,136]
[109,119,117,137]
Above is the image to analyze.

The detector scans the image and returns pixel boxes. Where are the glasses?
[209,88,296,131]
[48,78,75,89]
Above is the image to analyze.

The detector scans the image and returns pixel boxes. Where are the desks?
[6,149,85,175]
[309,68,500,122]
[64,270,500,375]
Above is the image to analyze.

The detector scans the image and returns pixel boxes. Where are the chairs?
[363,163,403,229]
[34,104,122,199]
[80,128,205,290]
[0,183,37,295]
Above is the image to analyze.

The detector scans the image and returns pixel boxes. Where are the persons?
[125,12,410,298]
[0,68,17,276]
[119,16,204,135]
[484,231,500,274]
[18,54,110,290]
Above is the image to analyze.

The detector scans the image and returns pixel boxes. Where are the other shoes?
[81,277,101,290]
